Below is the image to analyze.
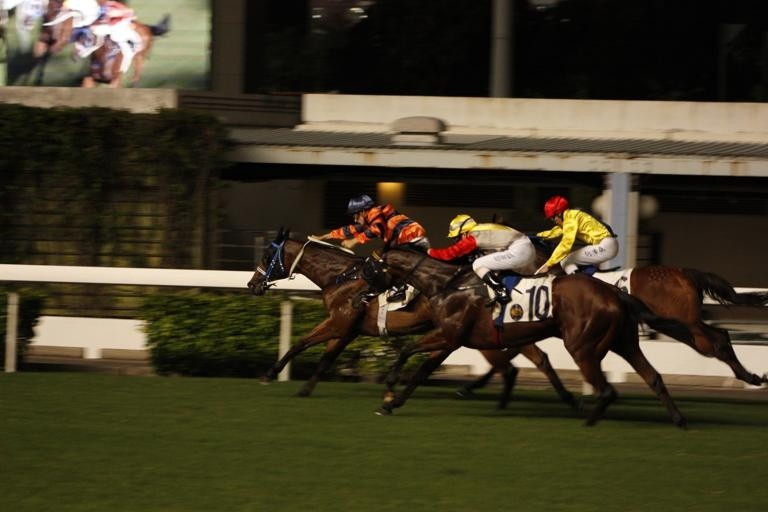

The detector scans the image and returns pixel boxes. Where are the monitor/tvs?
[0,1,211,89]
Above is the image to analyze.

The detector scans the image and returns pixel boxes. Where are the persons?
[309,194,430,302]
[531,195,618,278]
[425,214,537,307]
[70,0,145,73]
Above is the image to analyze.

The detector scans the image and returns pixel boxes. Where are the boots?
[386,280,407,302]
[482,271,511,307]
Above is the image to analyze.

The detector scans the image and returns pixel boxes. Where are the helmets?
[448,215,476,237]
[348,194,372,213]
[544,197,568,219]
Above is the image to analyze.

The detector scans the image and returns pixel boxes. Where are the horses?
[70,11,173,90]
[246,224,577,414]
[359,242,686,433]
[19,0,135,86]
[453,261,768,400]
